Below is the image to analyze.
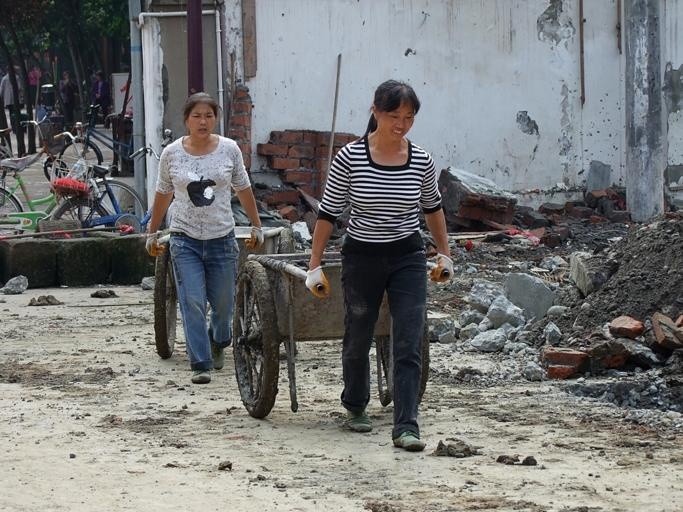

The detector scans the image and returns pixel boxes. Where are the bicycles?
[0,104,176,235]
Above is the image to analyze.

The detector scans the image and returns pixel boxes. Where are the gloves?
[245,226,264,249]
[145,233,167,259]
[304,267,330,299]
[430,253,455,282]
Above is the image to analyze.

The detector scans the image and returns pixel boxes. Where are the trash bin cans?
[41,84,53,108]
[107,118,133,176]
[4,104,27,133]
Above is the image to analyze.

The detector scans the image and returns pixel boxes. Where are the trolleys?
[232,251,450,420]
[154,224,304,360]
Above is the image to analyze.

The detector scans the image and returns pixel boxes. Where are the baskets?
[51,158,93,194]
[40,122,65,153]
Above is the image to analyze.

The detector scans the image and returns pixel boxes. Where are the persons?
[305,79,456,452]
[0,66,110,134]
[144,92,261,384]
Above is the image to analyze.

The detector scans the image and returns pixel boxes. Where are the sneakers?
[191,371,211,384]
[394,431,425,450]
[347,407,374,431]
[210,344,226,370]
